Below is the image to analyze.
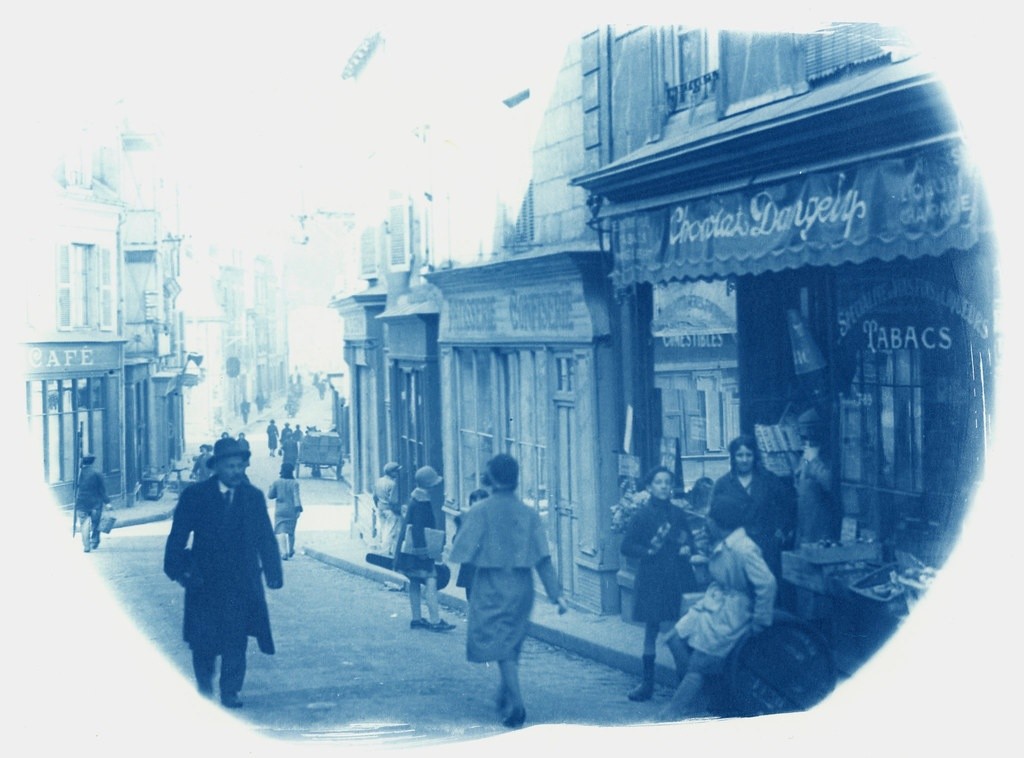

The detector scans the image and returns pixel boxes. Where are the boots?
[626,654,656,702]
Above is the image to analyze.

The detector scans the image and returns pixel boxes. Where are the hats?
[82,454,98,465]
[415,465,442,489]
[384,462,403,474]
[205,438,251,469]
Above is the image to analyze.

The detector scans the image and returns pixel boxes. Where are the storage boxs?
[782,537,927,618]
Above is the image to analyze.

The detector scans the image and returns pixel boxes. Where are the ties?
[223,491,231,521]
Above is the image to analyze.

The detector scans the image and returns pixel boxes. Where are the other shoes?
[83,547,90,552]
[219,692,243,708]
[410,618,457,633]
[281,555,288,560]
[289,550,295,558]
[91,545,98,550]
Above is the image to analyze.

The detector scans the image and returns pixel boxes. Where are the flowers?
[611,486,651,533]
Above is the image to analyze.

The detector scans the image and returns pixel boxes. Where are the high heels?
[502,707,527,730]
[492,689,506,711]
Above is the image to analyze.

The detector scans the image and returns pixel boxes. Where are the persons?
[372,461,403,556]
[164,438,283,708]
[620,405,843,701]
[267,463,302,561]
[646,500,776,723]
[189,432,245,481]
[391,465,455,632]
[452,489,489,604]
[448,453,568,728]
[240,397,250,425]
[73,456,112,552]
[267,419,340,472]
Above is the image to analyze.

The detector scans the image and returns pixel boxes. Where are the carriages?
[610,487,937,717]
[294,425,346,479]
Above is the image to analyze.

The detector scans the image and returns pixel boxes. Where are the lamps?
[180,352,202,380]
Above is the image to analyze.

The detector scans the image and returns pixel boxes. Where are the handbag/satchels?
[278,450,282,456]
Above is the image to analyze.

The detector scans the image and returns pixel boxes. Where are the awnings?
[598,132,980,290]
